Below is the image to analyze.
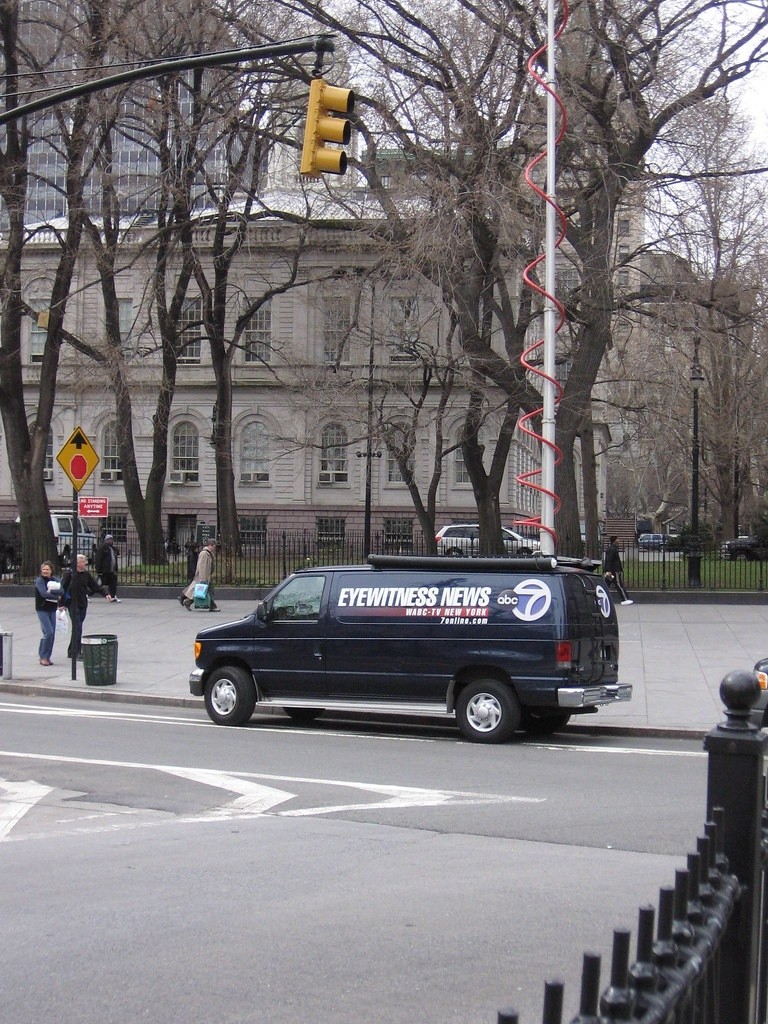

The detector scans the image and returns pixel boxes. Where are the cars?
[0,518,24,578]
[721,535,768,563]
[638,534,674,553]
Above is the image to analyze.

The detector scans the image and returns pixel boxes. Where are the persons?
[177,538,221,612]
[35,561,64,665]
[603,536,633,606]
[59,554,112,661]
[165,537,181,562]
[95,535,121,603]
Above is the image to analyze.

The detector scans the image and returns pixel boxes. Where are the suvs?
[14,509,100,568]
[186,554,635,745]
[434,523,542,559]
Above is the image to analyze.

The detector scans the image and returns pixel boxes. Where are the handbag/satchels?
[194,584,209,599]
[55,607,72,634]
[61,591,71,607]
[194,593,210,608]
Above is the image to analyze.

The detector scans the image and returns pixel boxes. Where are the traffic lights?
[300,79,355,180]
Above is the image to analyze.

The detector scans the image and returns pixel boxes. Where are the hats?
[206,538,219,545]
[610,536,617,543]
[105,535,113,540]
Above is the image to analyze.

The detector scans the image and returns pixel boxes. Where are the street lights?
[362,263,381,559]
[686,363,706,592]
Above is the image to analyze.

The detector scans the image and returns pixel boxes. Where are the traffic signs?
[78,497,108,518]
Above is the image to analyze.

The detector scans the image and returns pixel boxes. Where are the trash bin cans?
[81,634,118,686]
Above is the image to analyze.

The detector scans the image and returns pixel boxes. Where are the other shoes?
[184,600,191,611]
[621,600,633,606]
[48,660,53,665]
[112,596,121,603]
[177,596,184,606]
[39,658,49,666]
[68,652,83,660]
[87,595,92,603]
[209,608,220,612]
